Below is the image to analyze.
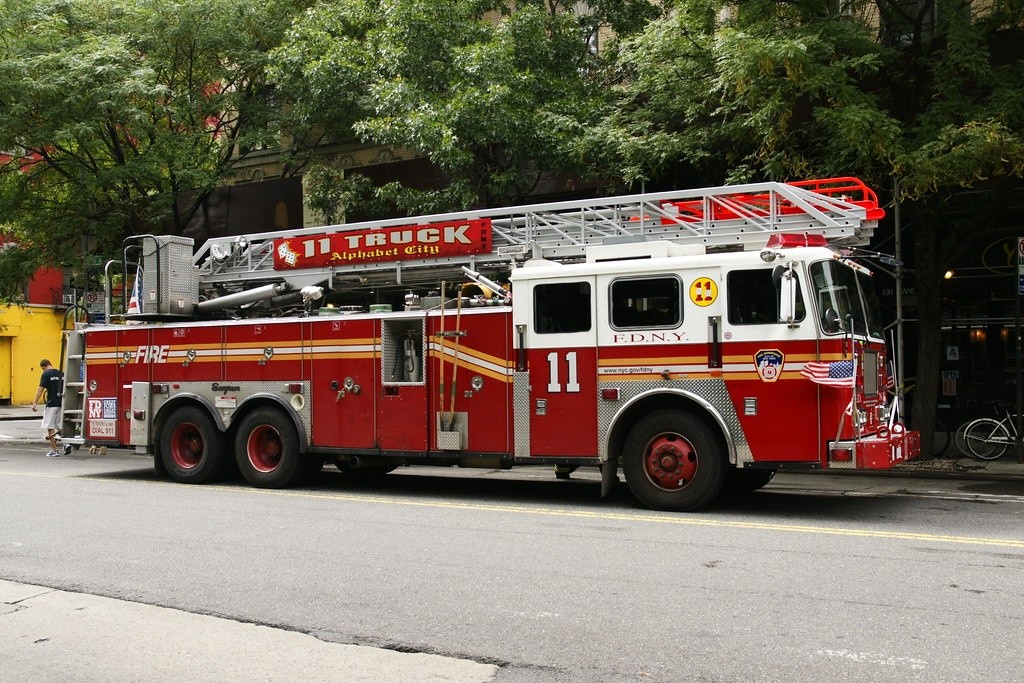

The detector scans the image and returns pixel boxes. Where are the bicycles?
[932,399,1024,460]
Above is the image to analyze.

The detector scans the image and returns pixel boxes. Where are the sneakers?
[46,450,59,456]
[63,444,71,455]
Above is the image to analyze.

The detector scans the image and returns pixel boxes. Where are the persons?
[32,358,71,457]
[88,445,107,455]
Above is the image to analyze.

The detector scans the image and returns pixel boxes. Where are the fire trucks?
[57,169,921,514]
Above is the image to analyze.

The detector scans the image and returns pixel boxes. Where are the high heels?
[100,447,106,455]
[89,446,96,454]
